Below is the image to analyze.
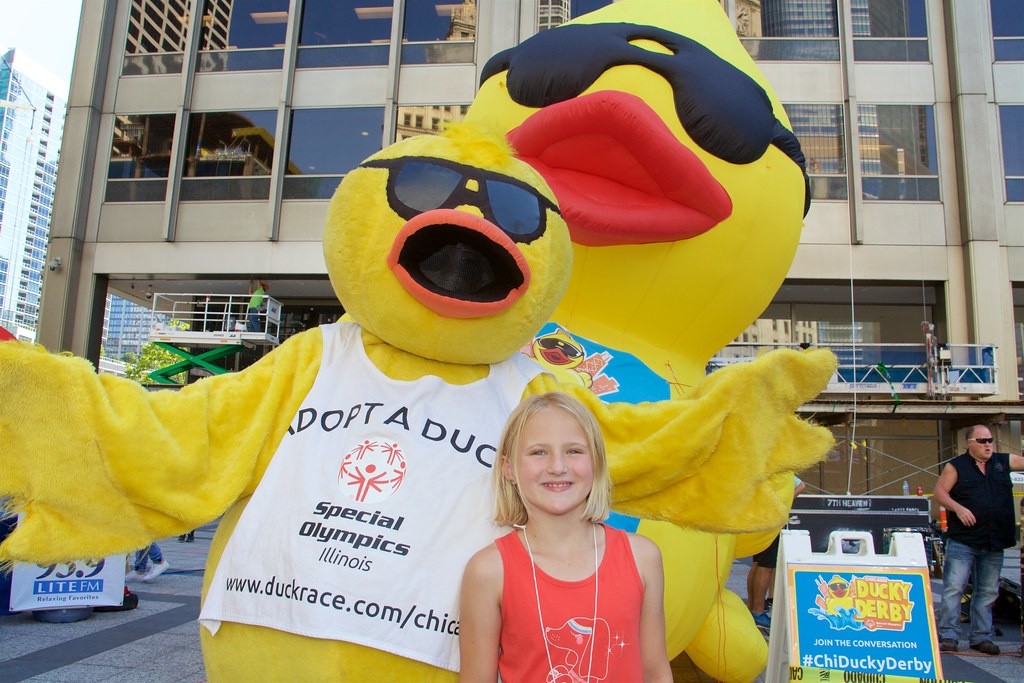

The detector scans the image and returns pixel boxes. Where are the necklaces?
[976,459,986,464]
[514,522,598,683]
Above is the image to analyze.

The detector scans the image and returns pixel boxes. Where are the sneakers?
[751,611,770,630]
[143,559,169,582]
[941,638,958,651]
[186,537,194,542]
[970,640,1000,655]
[178,538,185,543]
[125,570,148,582]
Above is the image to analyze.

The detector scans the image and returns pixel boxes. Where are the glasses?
[413,243,515,291]
[967,438,994,444]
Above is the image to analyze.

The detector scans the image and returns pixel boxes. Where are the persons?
[125,544,169,582]
[178,530,195,542]
[936,425,1024,653]
[459,393,673,683]
[748,475,806,629]
[247,275,269,332]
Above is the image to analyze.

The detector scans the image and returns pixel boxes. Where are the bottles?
[902,481,909,496]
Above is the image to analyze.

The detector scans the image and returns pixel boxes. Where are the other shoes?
[764,599,770,610]
[767,597,773,604]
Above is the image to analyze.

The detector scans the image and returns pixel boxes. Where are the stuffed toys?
[463,0,812,683]
[0,123,838,683]
